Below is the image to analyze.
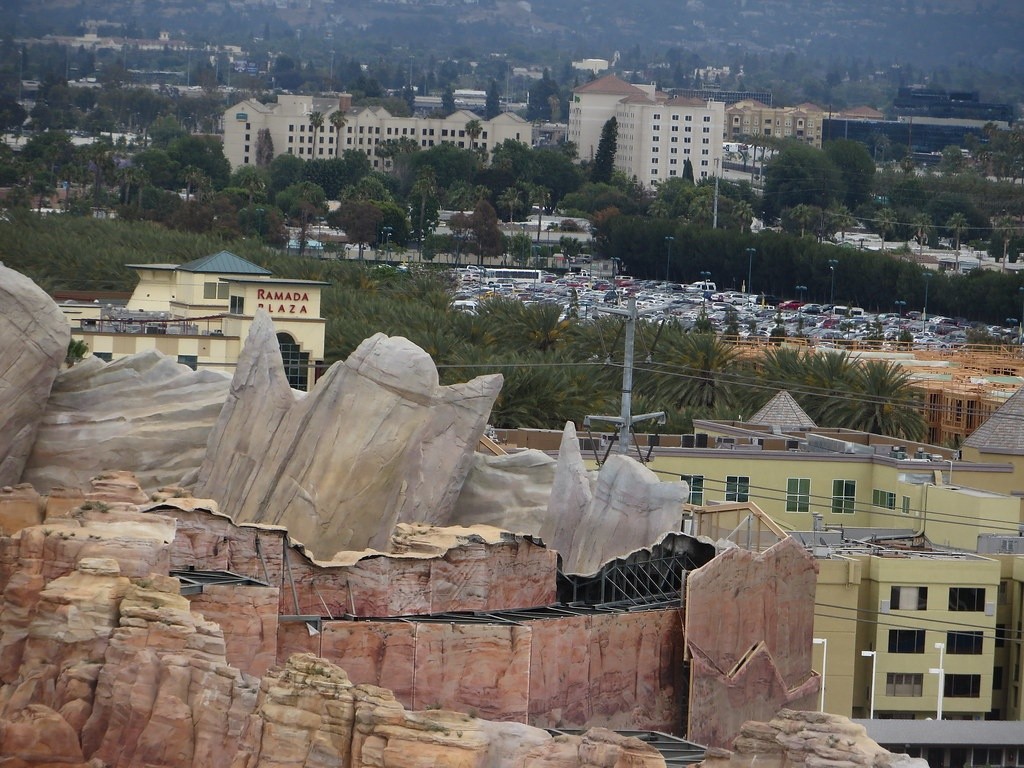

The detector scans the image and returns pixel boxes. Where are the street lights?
[746,248,756,309]
[383,226,393,273]
[928,642,945,720]
[894,300,907,342]
[861,649,875,719]
[828,259,838,319]
[609,257,620,308]
[794,286,808,336]
[448,263,1024,356]
[700,271,711,321]
[664,236,674,292]
[531,245,542,296]
[813,637,827,713]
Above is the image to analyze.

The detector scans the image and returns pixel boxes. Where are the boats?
[922,272,932,335]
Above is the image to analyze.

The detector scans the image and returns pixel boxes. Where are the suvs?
[574,254,593,264]
[723,293,749,306]
[756,294,778,306]
